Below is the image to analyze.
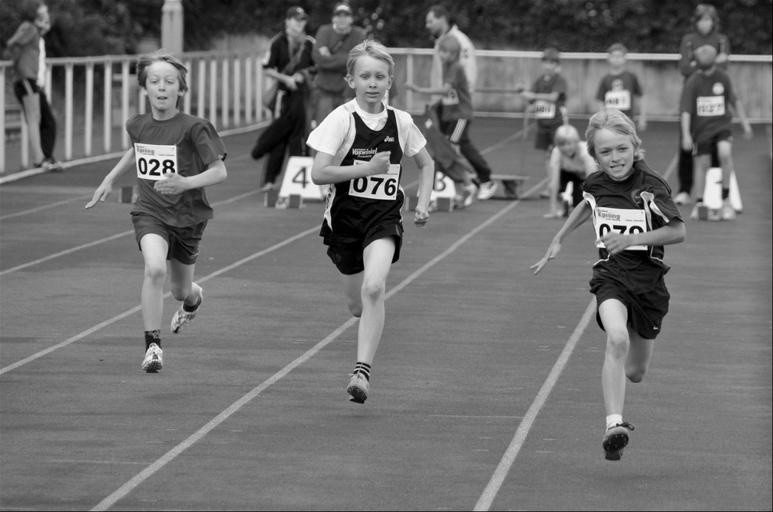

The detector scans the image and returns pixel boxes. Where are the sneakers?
[170,282,204,334]
[32,159,62,172]
[141,342,164,373]
[344,371,371,404]
[673,190,745,224]
[459,180,498,209]
[602,420,635,461]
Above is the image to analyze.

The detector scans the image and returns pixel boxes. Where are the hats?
[286,5,311,20]
[541,46,559,62]
[333,2,354,15]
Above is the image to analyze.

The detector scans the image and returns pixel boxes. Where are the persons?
[252,5,316,208]
[405,5,497,209]
[4,0,66,171]
[675,3,755,221]
[528,107,687,461]
[540,124,598,219]
[311,2,368,125]
[302,38,435,408]
[592,43,645,132]
[84,49,229,374]
[518,47,567,198]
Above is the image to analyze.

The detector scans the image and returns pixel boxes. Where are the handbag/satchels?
[261,80,281,106]
